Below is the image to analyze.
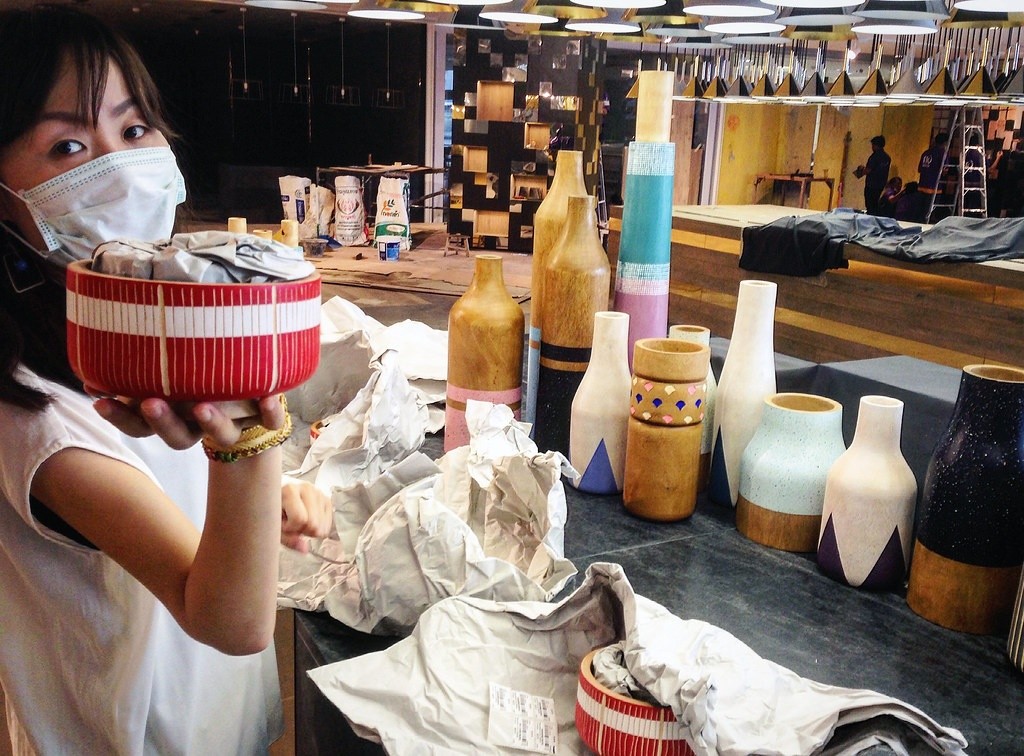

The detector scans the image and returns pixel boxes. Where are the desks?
[293,428,1024,756]
[317,165,448,224]
[754,174,835,213]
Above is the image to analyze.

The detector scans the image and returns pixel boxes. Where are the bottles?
[525,149,588,423]
[669,324,717,494]
[906,363,1024,635]
[533,193,612,461]
[736,391,846,551]
[444,254,526,453]
[816,394,917,591]
[570,310,632,491]
[704,280,778,507]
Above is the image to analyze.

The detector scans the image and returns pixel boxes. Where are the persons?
[856,129,1024,224]
[0,3,333,756]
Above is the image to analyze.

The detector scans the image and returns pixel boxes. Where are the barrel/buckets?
[301,238,329,260]
[376,235,401,261]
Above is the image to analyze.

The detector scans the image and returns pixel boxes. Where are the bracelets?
[201,392,292,463]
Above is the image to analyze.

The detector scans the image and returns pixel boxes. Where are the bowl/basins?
[65,259,322,399]
[574,648,696,756]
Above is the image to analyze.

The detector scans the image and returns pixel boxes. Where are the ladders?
[923,106,988,221]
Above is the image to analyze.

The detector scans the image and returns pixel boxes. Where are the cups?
[623,337,711,520]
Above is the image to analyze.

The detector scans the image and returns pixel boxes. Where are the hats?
[871,135,885,147]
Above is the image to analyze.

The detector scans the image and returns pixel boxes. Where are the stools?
[444,235,471,257]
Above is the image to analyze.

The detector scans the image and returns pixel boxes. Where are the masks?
[1,146,187,269]
